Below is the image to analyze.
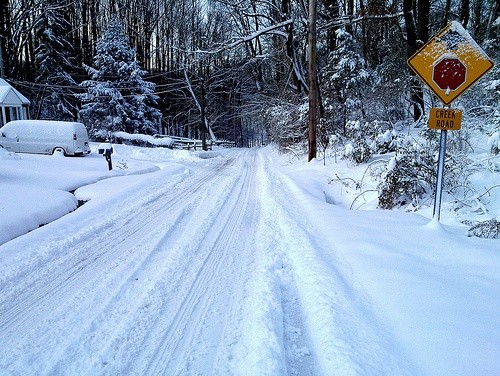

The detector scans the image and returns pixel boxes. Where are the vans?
[0,119,91,155]
